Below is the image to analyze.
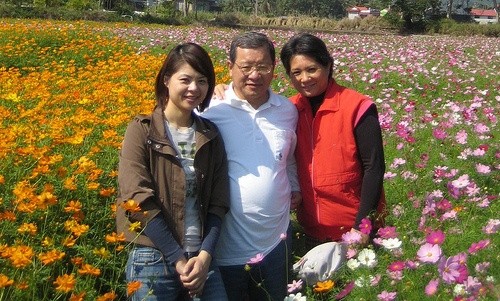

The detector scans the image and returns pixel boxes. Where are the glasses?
[234,62,274,74]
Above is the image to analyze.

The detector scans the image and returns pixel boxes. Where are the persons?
[118,42,230,301]
[212,33,389,260]
[193,32,300,300]
